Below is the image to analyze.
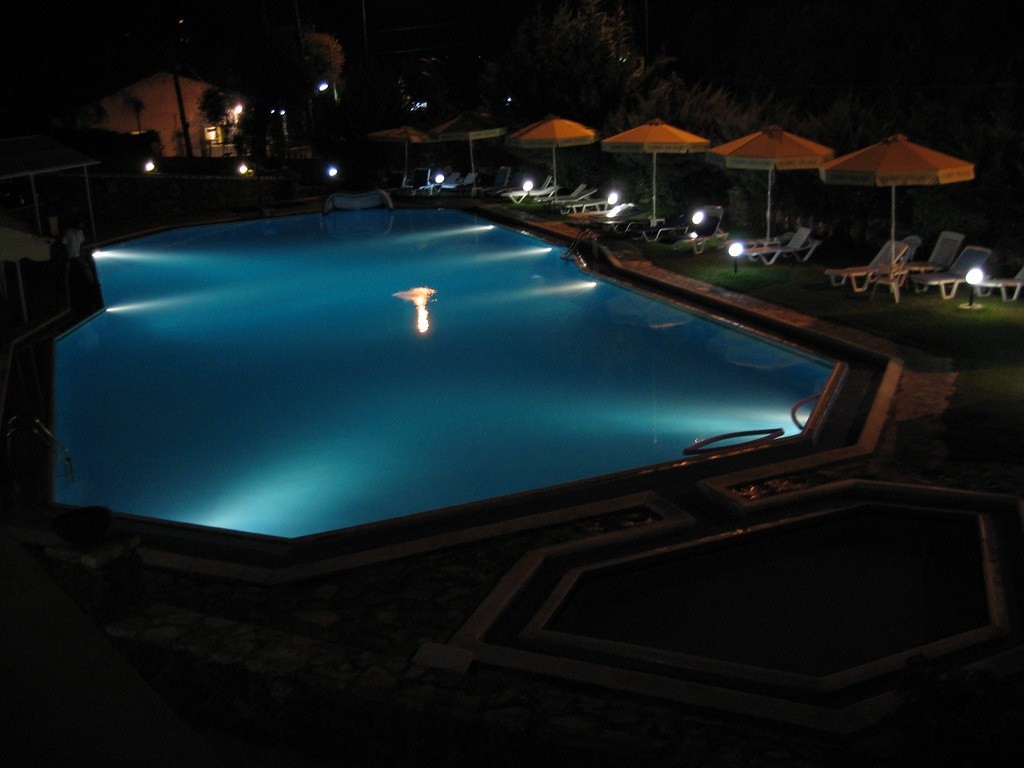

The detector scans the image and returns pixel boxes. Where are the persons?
[60,216,86,289]
[969,245,1021,305]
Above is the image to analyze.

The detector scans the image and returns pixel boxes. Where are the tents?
[0,206,51,324]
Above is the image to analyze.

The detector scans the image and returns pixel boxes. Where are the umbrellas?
[429,113,508,174]
[366,127,439,181]
[706,126,835,240]
[602,118,710,227]
[506,114,600,204]
[820,133,975,283]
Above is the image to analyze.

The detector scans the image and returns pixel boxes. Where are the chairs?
[389,165,822,265]
[824,230,1024,304]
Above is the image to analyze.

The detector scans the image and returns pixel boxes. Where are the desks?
[644,216,665,228]
[472,187,482,196]
